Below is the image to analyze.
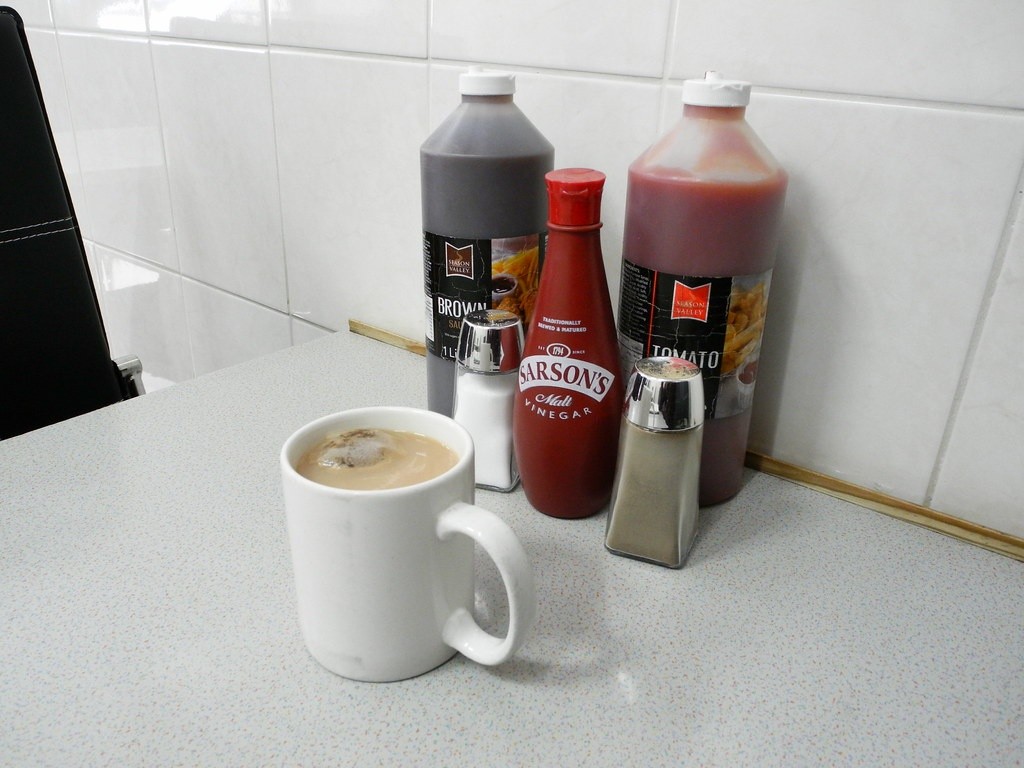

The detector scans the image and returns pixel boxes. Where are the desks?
[0,329,1024,768]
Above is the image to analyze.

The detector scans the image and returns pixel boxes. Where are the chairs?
[0,6,148,443]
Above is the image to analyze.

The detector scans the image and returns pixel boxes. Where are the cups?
[281,403,532,680]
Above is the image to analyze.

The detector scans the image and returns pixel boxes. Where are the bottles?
[449,306,526,495]
[613,69,792,509]
[512,167,622,520]
[416,73,556,460]
[603,357,708,568]
